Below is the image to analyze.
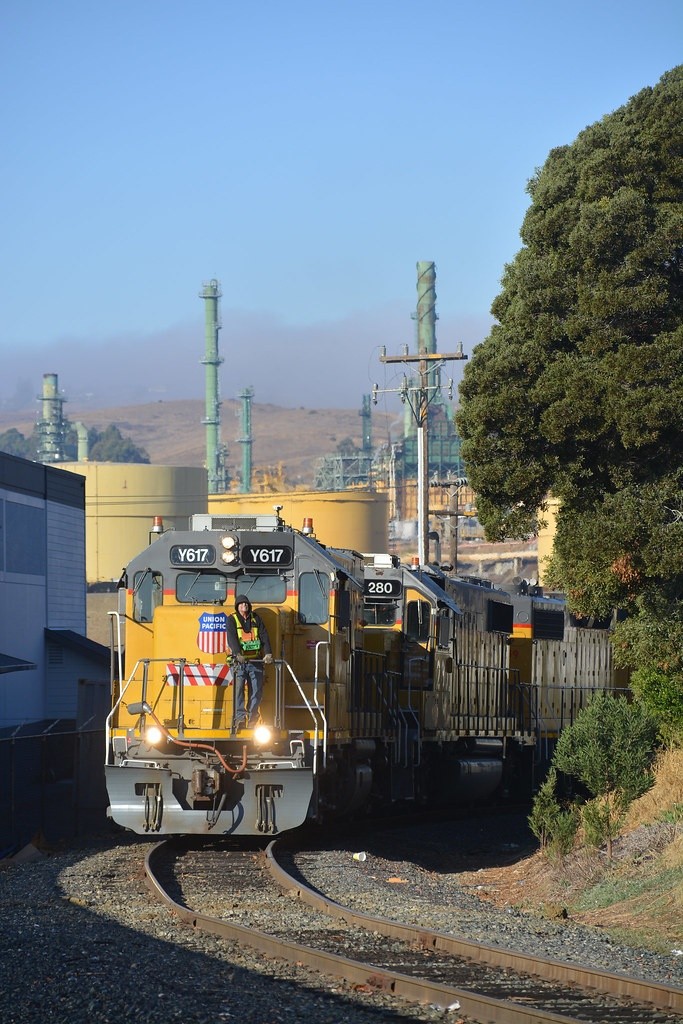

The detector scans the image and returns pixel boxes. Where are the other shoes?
[236,721,244,734]
[247,722,255,729]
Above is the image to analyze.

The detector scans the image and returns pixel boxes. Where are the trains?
[103,505,619,837]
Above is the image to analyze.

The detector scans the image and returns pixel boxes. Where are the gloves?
[237,653,245,664]
[263,654,274,664]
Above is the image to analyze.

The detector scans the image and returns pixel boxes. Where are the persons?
[226,594,273,729]
